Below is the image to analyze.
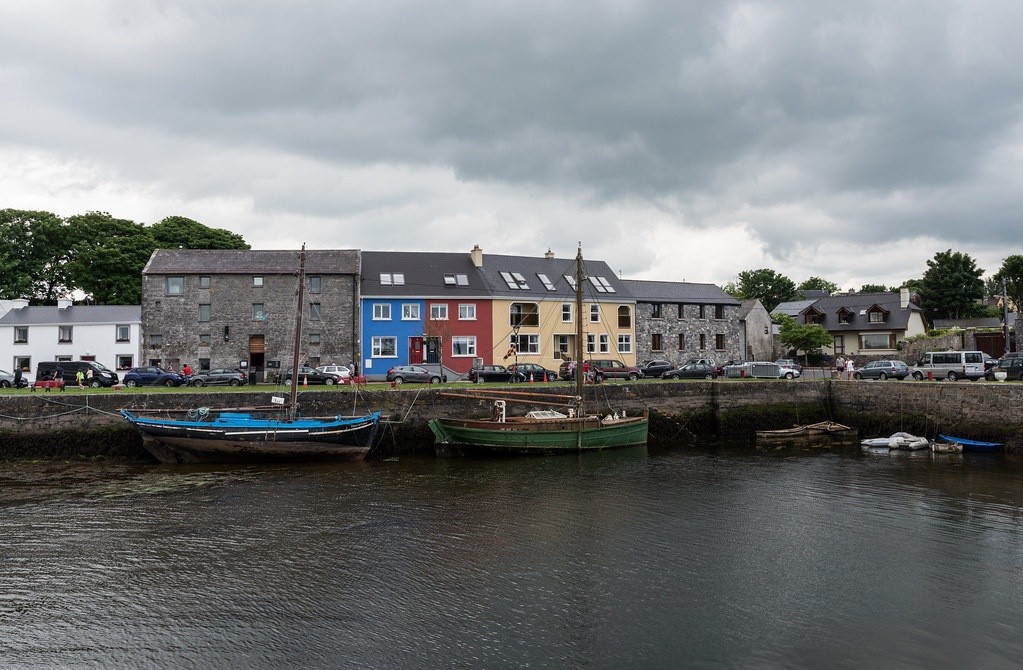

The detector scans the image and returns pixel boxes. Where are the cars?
[0,369,28,389]
[983,353,998,370]
[273,366,340,386]
[717,360,801,380]
[984,351,1023,382]
[662,363,718,381]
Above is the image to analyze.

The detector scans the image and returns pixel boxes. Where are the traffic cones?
[302,374,307,387]
[543,371,547,382]
[530,372,535,384]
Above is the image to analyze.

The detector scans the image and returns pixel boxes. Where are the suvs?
[316,366,351,379]
[559,360,584,381]
[686,358,717,369]
[190,366,247,388]
[585,360,644,383]
[635,359,674,378]
[469,364,527,384]
[507,363,557,382]
[122,366,185,387]
[386,366,447,385]
[853,359,910,380]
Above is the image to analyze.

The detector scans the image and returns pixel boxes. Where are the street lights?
[513,324,520,383]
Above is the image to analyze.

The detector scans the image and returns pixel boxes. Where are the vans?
[35,361,119,388]
[911,351,986,382]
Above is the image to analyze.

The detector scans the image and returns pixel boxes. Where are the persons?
[574,364,577,383]
[304,361,310,371]
[53,364,64,381]
[349,361,358,387]
[846,356,855,380]
[836,356,845,380]
[15,365,23,389]
[583,361,604,384]
[183,365,191,386]
[1006,347,1009,353]
[86,367,93,388]
[167,361,172,371]
[948,347,956,358]
[76,369,84,389]
[156,363,163,370]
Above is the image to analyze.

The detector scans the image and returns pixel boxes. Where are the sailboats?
[429,240,651,453]
[114,242,405,460]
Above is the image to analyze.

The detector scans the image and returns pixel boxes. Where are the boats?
[938,434,1007,454]
[861,431,930,450]
[754,420,859,438]
